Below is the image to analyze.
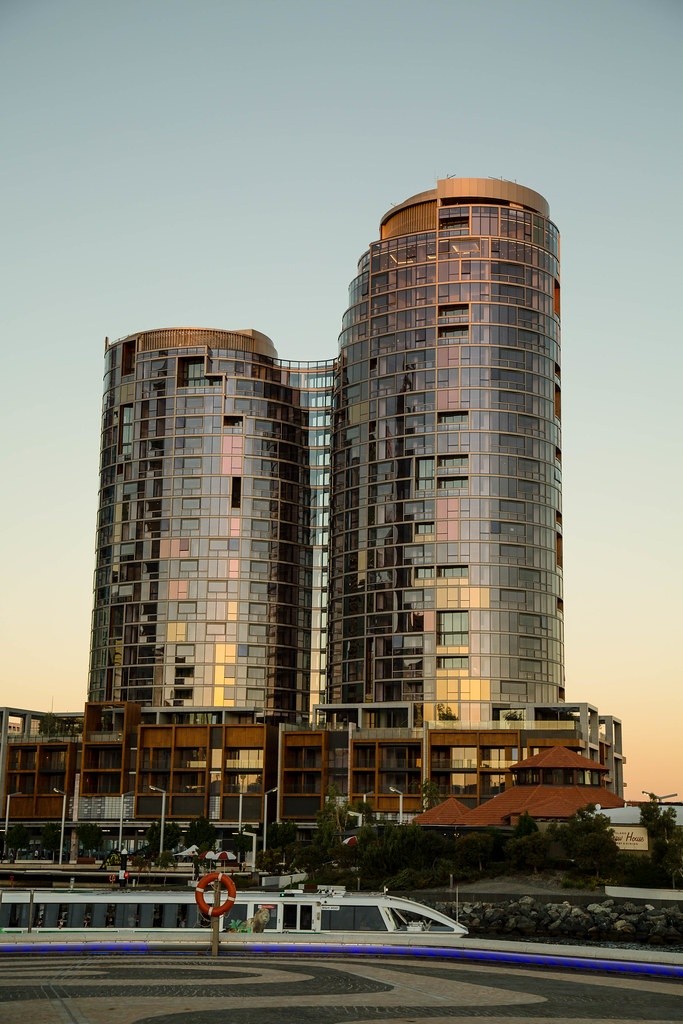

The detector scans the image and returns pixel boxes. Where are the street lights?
[148,785,167,857]
[363,791,374,803]
[263,787,279,852]
[53,787,67,865]
[119,790,135,852]
[389,786,403,824]
[2,792,22,856]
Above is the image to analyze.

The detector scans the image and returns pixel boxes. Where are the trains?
[0,884,470,939]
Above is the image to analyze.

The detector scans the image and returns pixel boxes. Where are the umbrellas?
[199,850,215,874]
[212,851,237,874]
[343,836,359,845]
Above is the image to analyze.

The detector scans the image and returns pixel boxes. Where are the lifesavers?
[109,874,116,882]
[195,873,236,917]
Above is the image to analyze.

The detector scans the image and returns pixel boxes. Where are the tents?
[172,845,201,881]
